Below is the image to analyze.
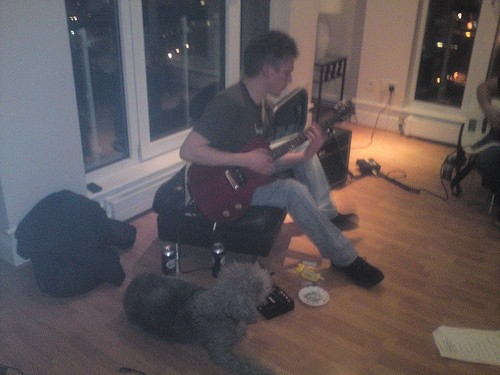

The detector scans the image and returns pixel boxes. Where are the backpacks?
[15,189,137,299]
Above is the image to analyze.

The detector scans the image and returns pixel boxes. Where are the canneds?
[211,243,225,278]
[161,246,177,275]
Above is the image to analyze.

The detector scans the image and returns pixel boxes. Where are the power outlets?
[388,83,396,97]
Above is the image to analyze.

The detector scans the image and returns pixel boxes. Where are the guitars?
[459,99,500,155]
[187,98,354,226]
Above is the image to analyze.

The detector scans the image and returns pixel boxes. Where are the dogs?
[119,259,274,371]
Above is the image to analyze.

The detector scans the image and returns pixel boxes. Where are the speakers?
[317,124,351,189]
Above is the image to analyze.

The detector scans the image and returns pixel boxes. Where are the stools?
[153,164,289,256]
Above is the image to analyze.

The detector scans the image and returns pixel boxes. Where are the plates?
[298,287,329,306]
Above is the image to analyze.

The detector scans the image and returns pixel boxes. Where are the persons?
[180,30,384,287]
[476,74,500,226]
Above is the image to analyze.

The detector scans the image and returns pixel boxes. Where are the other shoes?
[331,255,384,289]
[447,141,500,172]
[329,213,360,231]
